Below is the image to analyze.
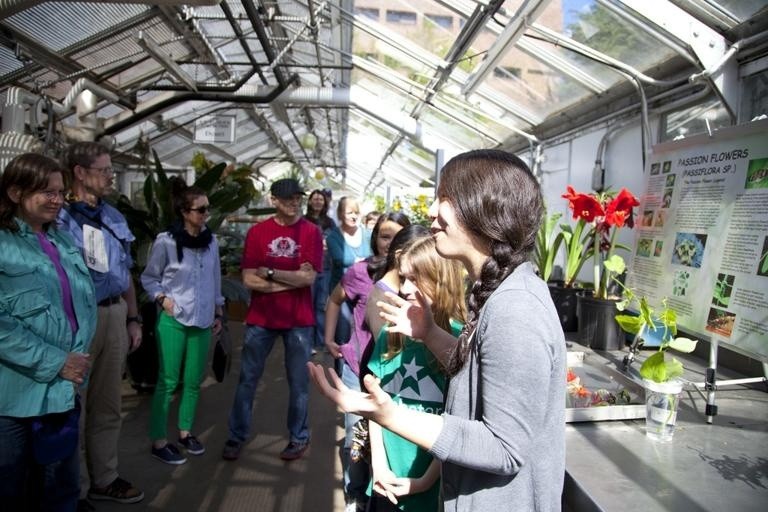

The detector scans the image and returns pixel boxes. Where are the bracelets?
[215,314,224,320]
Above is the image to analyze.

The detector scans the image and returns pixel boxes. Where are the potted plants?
[641,293,700,441]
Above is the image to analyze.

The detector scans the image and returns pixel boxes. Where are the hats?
[270,179,307,198]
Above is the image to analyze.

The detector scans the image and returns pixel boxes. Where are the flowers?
[531,185,659,332]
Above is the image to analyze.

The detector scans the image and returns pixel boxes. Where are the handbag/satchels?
[351,417,371,468]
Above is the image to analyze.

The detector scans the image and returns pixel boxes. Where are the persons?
[363,233,468,512]
[306,148,569,512]
[222,178,323,461]
[1,153,99,512]
[140,188,225,466]
[304,190,470,512]
[54,142,144,504]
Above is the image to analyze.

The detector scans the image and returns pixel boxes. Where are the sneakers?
[89,477,144,504]
[177,433,205,456]
[151,442,188,464]
[279,442,309,459]
[222,439,241,461]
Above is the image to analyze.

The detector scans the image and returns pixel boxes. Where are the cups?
[643,378,683,442]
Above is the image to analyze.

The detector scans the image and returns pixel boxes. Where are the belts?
[99,294,121,306]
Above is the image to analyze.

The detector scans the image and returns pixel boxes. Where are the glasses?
[44,192,65,199]
[84,166,115,175]
[191,205,210,213]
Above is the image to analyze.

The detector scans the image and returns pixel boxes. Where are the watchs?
[127,315,143,323]
[268,267,274,280]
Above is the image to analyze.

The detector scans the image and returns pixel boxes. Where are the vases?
[548,275,631,351]
